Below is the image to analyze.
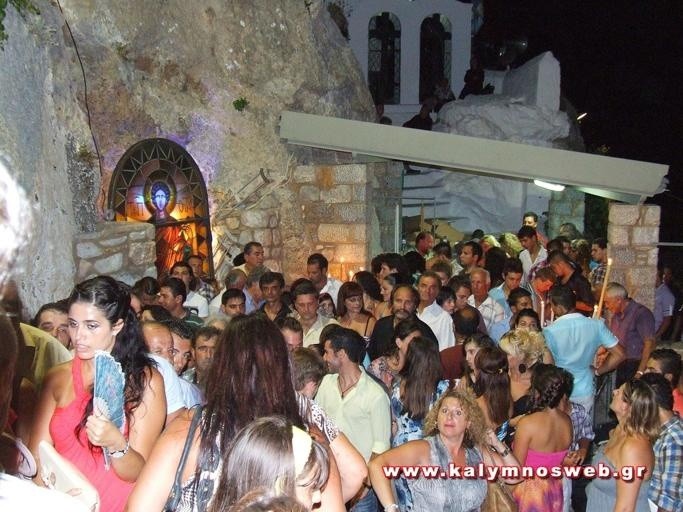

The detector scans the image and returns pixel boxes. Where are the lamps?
[532,178,571,193]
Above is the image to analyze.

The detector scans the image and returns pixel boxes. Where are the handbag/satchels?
[481,480,519,512]
[37,439,102,512]
